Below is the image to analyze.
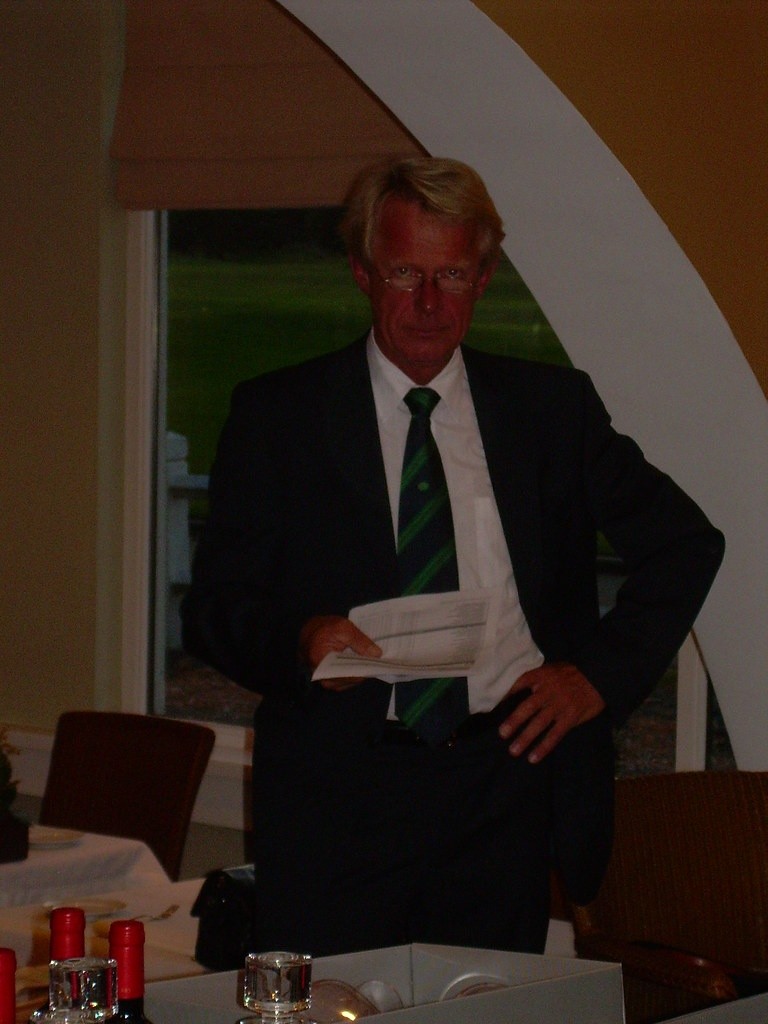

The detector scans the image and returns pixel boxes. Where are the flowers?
[0,724,22,814]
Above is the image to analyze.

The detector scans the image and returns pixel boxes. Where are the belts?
[381,715,498,751]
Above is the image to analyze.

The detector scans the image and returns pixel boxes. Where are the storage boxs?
[141,941,626,1024]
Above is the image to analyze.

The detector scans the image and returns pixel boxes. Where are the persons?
[178,153,728,960]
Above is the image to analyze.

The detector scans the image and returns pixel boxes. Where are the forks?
[98,903,180,937]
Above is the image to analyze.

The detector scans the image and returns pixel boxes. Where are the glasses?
[367,263,482,293]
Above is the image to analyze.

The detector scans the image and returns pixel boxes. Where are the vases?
[0,815,32,863]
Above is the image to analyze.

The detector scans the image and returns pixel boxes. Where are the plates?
[29,828,83,850]
[43,898,127,922]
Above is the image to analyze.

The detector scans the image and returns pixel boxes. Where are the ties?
[394,388,470,748]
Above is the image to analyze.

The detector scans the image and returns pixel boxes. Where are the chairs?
[37,710,217,884]
[567,769,768,1024]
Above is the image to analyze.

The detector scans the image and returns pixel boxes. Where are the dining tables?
[0,823,171,907]
[0,876,256,986]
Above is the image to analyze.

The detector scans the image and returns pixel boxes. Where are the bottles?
[0,948,21,1024]
[101,919,153,1024]
[31,908,88,1024]
